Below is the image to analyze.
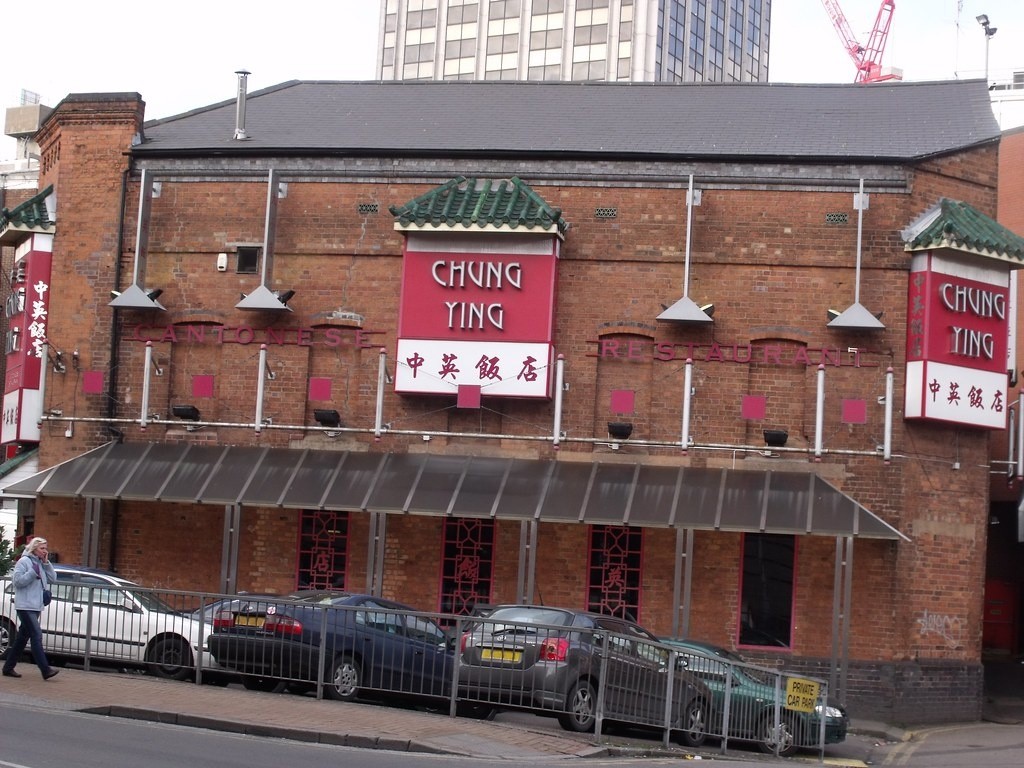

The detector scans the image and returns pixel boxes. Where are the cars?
[0,569,228,687]
[206,591,497,722]
[450,603,714,749]
[657,636,851,757]
[176,593,302,621]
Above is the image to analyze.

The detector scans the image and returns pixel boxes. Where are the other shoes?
[2,668,22,677]
[42,668,59,679]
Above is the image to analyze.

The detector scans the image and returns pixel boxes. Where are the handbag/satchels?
[43,589,52,606]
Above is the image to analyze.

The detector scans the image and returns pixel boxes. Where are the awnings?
[0,441,913,543]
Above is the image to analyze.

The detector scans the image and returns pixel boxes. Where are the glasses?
[38,547,48,550]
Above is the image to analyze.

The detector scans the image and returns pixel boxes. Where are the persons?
[2,537,59,680]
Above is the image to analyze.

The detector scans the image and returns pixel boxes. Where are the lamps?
[607,421,633,449]
[763,429,788,458]
[313,409,342,437]
[173,405,200,430]
